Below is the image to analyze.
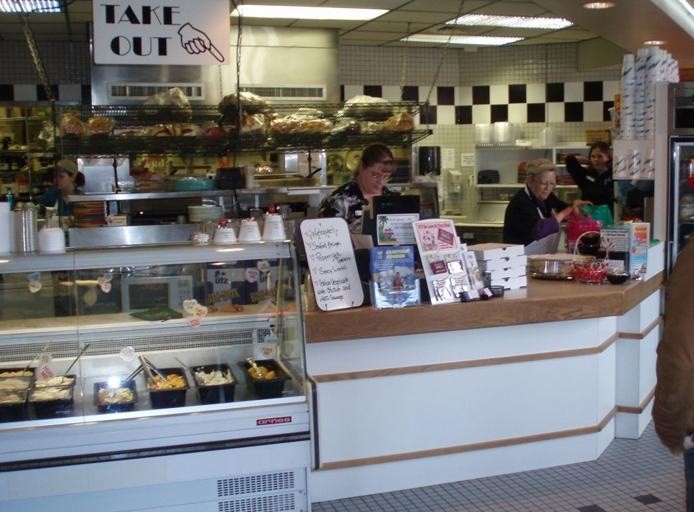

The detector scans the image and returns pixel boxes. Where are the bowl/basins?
[214,166,248,190]
[165,174,214,192]
[605,272,631,286]
[531,259,573,276]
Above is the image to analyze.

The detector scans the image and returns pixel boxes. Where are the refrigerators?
[665,134,693,281]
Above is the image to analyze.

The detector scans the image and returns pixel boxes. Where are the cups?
[608,43,683,177]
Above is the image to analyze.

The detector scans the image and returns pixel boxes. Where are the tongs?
[139,354,174,388]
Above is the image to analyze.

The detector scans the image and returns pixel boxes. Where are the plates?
[344,149,364,173]
[186,205,225,224]
[0,201,106,253]
[326,154,345,172]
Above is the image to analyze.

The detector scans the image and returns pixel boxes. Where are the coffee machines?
[445,169,464,215]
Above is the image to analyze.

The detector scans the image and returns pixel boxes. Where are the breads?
[60,87,416,135]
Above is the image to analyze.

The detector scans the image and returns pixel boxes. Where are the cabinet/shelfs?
[473,141,613,207]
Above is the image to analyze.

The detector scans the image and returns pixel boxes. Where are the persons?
[37,159,93,318]
[650,232,693,511]
[564,141,614,217]
[316,142,401,234]
[502,157,594,255]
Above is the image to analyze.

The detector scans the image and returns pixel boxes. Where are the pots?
[471,120,522,148]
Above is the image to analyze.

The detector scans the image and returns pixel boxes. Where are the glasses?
[370,173,394,183]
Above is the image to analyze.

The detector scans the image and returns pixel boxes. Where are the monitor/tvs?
[359,209,377,263]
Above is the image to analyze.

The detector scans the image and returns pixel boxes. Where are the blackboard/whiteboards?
[300,217,365,312]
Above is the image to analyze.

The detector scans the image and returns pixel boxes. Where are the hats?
[57,158,79,176]
[526,159,558,176]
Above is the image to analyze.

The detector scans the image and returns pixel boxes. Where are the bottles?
[679,158,694,222]
[213,228,236,243]
[262,213,287,241]
[6,187,15,206]
[541,122,560,148]
[237,220,261,242]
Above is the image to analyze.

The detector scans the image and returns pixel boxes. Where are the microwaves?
[119,273,195,313]
